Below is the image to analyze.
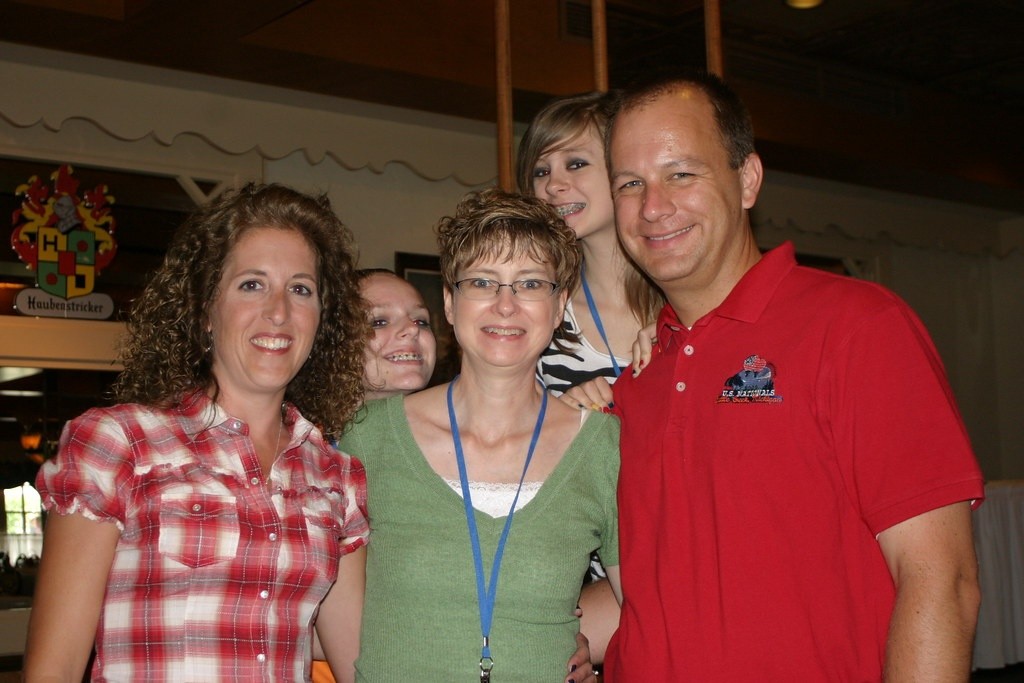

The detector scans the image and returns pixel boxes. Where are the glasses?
[455,277,558,301]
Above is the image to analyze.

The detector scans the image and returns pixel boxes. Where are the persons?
[311,186,619,683]
[516,89,673,582]
[24,182,371,683]
[579,71,986,683]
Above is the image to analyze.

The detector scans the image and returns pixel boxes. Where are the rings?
[592,671,599,677]
